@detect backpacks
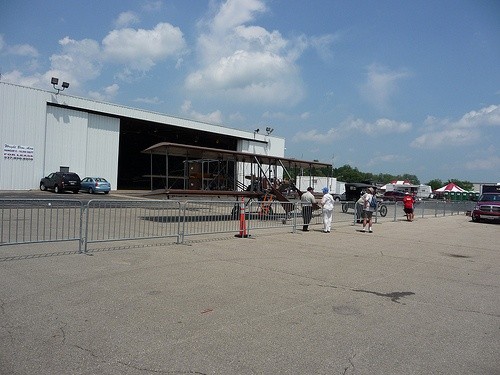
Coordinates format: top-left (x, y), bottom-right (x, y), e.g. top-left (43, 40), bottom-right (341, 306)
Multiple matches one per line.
top-left (370, 197), bottom-right (377, 207)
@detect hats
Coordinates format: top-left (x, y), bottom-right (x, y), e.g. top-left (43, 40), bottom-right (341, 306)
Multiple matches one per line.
top-left (405, 192), bottom-right (409, 195)
top-left (368, 188), bottom-right (374, 194)
top-left (322, 188), bottom-right (328, 193)
top-left (307, 187), bottom-right (313, 191)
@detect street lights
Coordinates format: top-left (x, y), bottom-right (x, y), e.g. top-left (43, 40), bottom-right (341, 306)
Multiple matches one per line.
top-left (253, 129), bottom-right (260, 176)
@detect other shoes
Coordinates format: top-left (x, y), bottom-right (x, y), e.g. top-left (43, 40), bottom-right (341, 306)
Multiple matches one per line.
top-left (321, 230), bottom-right (327, 233)
top-left (360, 230), bottom-right (365, 232)
top-left (370, 231), bottom-right (372, 232)
top-left (327, 231), bottom-right (330, 233)
top-left (304, 230), bottom-right (309, 232)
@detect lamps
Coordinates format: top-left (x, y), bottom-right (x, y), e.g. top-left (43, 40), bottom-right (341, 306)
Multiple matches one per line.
top-left (266, 127), bottom-right (270, 133)
top-left (61, 82), bottom-right (70, 90)
top-left (51, 77), bottom-right (59, 88)
top-left (270, 128), bottom-right (274, 133)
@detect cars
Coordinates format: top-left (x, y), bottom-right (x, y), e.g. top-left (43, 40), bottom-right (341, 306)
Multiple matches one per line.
top-left (471, 193), bottom-right (500, 223)
top-left (383, 191), bottom-right (413, 202)
top-left (79, 177), bottom-right (111, 194)
top-left (341, 183), bottom-right (388, 217)
top-left (333, 192), bottom-right (347, 202)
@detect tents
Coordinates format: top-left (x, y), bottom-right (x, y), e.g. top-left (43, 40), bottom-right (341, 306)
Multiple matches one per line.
top-left (434, 182), bottom-right (467, 195)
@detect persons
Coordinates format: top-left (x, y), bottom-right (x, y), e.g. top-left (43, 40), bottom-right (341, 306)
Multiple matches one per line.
top-left (360, 187), bottom-right (376, 232)
top-left (403, 192), bottom-right (415, 222)
top-left (300, 186), bottom-right (319, 231)
top-left (321, 188), bottom-right (334, 233)
top-left (357, 197), bottom-right (364, 222)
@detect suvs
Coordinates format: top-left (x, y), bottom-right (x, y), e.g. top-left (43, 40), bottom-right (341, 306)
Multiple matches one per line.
top-left (40, 172), bottom-right (82, 194)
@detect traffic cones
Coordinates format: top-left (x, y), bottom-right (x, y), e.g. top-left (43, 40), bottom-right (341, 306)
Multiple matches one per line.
top-left (234, 197), bottom-right (252, 238)
